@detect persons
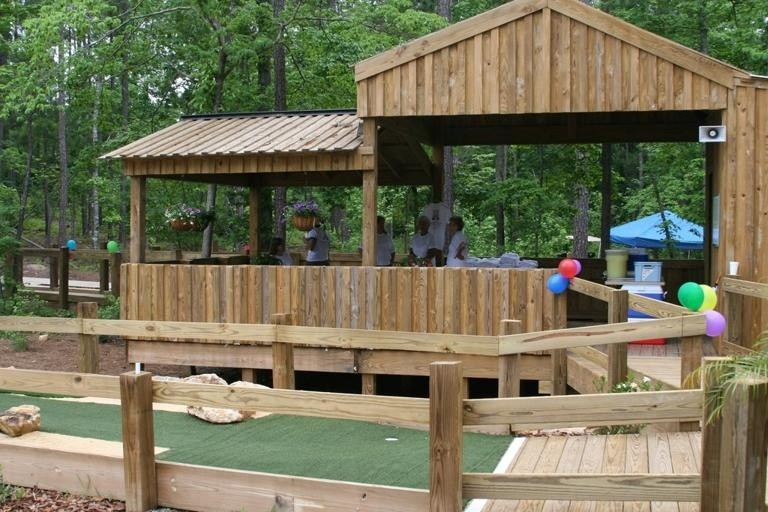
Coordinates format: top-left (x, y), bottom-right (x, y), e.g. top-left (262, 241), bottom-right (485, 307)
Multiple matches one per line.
top-left (269, 215), bottom-right (470, 266)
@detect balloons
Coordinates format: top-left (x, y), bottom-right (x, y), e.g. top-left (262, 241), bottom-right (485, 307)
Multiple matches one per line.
top-left (546, 258), bottom-right (582, 295)
top-left (66, 239), bottom-right (77, 251)
top-left (677, 281), bottom-right (727, 338)
top-left (106, 240), bottom-right (120, 253)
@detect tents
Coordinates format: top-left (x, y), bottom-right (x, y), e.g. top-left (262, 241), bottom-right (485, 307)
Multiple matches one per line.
top-left (608, 209), bottom-right (720, 260)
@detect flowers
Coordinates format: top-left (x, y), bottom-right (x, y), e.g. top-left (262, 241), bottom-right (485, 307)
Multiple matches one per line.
top-left (282, 199), bottom-right (326, 223)
top-left (164, 199), bottom-right (214, 224)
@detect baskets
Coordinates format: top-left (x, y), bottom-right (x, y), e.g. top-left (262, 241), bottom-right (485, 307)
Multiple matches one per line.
top-left (170, 219), bottom-right (199, 231)
top-left (293, 215), bottom-right (319, 231)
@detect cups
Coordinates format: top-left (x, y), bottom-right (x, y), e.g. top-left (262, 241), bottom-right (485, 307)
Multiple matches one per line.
top-left (729, 262), bottom-right (739, 275)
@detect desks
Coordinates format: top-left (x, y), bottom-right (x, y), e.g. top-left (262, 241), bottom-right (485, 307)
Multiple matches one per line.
top-left (603, 270), bottom-right (666, 288)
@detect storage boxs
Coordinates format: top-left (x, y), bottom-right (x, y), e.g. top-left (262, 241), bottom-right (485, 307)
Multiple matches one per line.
top-left (619, 282), bottom-right (667, 317)
top-left (626, 315), bottom-right (668, 345)
top-left (634, 261), bottom-right (666, 284)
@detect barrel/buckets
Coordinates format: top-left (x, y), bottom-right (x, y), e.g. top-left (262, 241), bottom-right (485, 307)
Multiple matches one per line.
top-left (627, 248), bottom-right (648, 276)
top-left (605, 250), bottom-right (628, 278)
